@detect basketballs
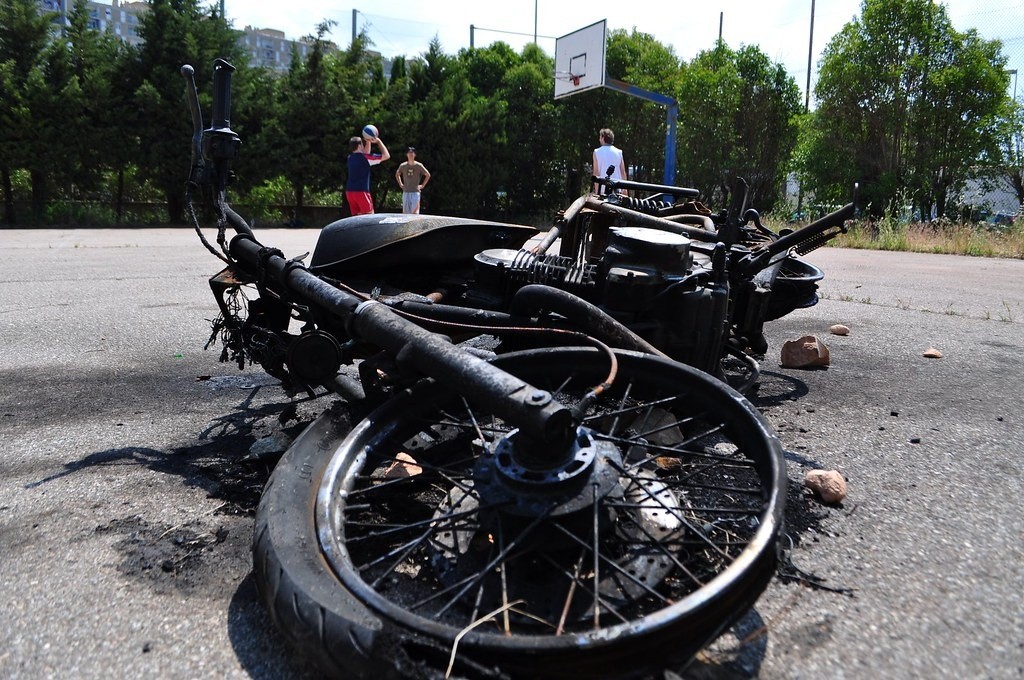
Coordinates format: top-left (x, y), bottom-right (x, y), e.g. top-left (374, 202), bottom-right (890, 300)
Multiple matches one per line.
top-left (362, 124), bottom-right (379, 140)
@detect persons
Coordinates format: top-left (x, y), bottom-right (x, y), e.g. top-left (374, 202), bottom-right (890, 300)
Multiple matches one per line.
top-left (346, 137), bottom-right (390, 215)
top-left (593, 128), bottom-right (627, 197)
top-left (395, 147), bottom-right (430, 215)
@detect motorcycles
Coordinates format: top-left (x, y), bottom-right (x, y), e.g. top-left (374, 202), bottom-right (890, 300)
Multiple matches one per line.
top-left (179, 52), bottom-right (857, 678)
